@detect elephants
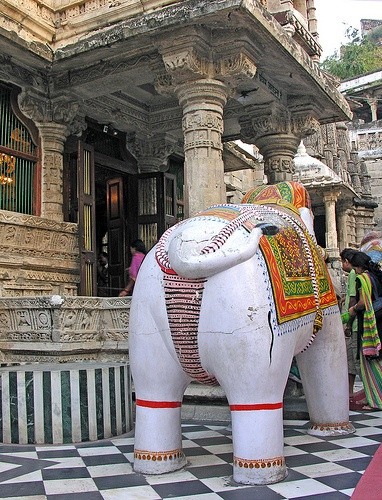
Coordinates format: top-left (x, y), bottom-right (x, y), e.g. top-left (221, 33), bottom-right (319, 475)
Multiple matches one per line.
top-left (128, 180), bottom-right (356, 485)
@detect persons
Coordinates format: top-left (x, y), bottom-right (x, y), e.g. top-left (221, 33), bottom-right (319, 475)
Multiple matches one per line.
top-left (348, 251), bottom-right (382, 411)
top-left (339, 248), bottom-right (369, 405)
top-left (97, 252), bottom-right (109, 297)
top-left (118, 238), bottom-right (146, 297)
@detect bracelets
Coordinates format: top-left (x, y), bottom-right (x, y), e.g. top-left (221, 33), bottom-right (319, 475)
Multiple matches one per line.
top-left (124, 288), bottom-right (129, 293)
top-left (345, 323), bottom-right (352, 330)
top-left (354, 304), bottom-right (359, 313)
